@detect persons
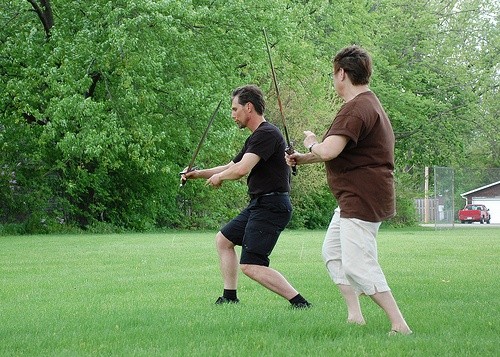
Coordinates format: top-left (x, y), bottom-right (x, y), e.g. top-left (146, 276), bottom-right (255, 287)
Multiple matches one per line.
top-left (181, 83), bottom-right (312, 312)
top-left (285, 47), bottom-right (412, 338)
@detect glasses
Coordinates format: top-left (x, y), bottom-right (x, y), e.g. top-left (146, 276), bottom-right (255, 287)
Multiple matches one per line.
top-left (328, 69), bottom-right (340, 80)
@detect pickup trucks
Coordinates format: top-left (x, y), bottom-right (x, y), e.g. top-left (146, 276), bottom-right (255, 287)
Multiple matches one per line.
top-left (458, 204), bottom-right (491, 224)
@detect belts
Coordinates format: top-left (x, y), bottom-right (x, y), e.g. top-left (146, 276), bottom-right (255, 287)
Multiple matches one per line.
top-left (263, 191), bottom-right (289, 196)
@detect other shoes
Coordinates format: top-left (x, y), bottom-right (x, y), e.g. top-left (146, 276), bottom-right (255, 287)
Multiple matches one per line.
top-left (294, 302), bottom-right (310, 309)
top-left (215, 296), bottom-right (239, 304)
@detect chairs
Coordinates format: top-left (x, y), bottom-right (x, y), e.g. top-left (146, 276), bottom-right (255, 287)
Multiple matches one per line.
top-left (468, 206), bottom-right (472, 209)
top-left (477, 207), bottom-right (481, 210)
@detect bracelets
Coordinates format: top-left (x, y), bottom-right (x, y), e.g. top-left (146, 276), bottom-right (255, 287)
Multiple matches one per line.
top-left (309, 142), bottom-right (317, 152)
